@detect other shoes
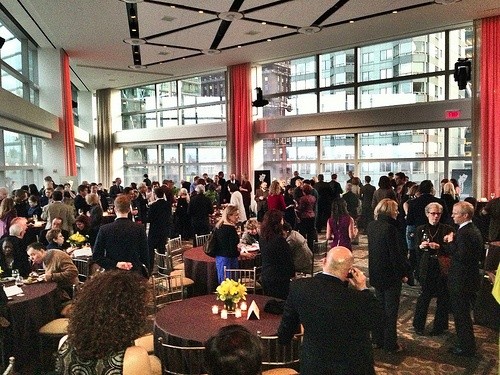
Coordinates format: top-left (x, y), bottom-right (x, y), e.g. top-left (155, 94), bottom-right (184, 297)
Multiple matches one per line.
top-left (449, 345), bottom-right (476, 355)
top-left (384, 343), bottom-right (408, 355)
top-left (414, 328), bottom-right (427, 336)
top-left (432, 324), bottom-right (449, 333)
top-left (371, 342), bottom-right (383, 349)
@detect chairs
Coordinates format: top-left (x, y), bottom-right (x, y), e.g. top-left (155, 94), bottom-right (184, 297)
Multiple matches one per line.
top-left (0, 232), bottom-right (329, 375)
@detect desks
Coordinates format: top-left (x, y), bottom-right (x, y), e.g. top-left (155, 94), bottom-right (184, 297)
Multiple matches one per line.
top-left (183, 244), bottom-right (260, 300)
top-left (0, 281), bottom-right (58, 369)
top-left (153, 294), bottom-right (301, 374)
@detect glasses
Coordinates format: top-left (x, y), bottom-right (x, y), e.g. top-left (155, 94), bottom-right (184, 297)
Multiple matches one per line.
top-left (428, 212), bottom-right (443, 216)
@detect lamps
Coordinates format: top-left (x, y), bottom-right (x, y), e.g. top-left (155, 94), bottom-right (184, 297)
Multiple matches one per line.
top-left (252, 87), bottom-right (269, 107)
top-left (454, 58), bottom-right (472, 89)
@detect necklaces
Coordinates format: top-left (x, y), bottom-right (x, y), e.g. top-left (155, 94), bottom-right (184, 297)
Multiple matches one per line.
top-left (428, 227), bottom-right (439, 241)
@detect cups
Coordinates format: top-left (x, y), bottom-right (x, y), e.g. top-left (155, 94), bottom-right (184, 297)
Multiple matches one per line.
top-left (33, 215), bottom-right (38, 224)
top-left (12, 268), bottom-right (18, 276)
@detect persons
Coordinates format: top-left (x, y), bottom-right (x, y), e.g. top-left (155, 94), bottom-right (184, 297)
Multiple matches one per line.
top-left (485, 262), bottom-right (500, 305)
top-left (0, 170), bottom-right (500, 316)
top-left (278, 246), bottom-right (387, 375)
top-left (201, 324), bottom-right (301, 375)
top-left (411, 201), bottom-right (456, 336)
top-left (52, 269), bottom-right (163, 375)
top-left (367, 198), bottom-right (414, 356)
top-left (427, 201), bottom-right (484, 356)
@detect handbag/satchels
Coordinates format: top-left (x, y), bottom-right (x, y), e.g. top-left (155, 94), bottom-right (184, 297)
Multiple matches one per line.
top-left (203, 228), bottom-right (220, 257)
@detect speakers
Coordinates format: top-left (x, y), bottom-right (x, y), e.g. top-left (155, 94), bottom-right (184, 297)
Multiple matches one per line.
top-left (254, 170), bottom-right (270, 195)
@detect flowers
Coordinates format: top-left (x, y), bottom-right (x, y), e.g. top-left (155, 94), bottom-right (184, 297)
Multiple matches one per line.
top-left (213, 278), bottom-right (248, 305)
